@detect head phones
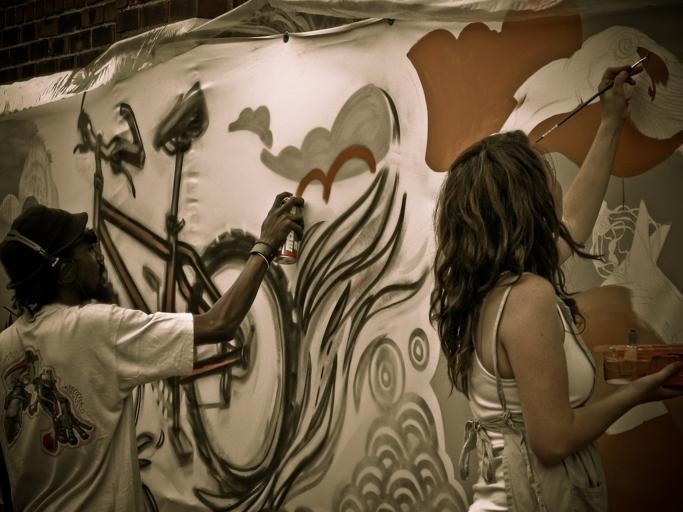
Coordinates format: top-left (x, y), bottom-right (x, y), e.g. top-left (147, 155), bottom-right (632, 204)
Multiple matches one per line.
top-left (4, 230), bottom-right (79, 289)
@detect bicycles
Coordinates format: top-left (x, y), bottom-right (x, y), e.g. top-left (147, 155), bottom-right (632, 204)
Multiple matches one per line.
top-left (73, 81), bottom-right (302, 494)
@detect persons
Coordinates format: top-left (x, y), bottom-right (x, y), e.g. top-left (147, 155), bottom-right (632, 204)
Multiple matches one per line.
top-left (428, 65), bottom-right (681, 511)
top-left (0, 191), bottom-right (306, 512)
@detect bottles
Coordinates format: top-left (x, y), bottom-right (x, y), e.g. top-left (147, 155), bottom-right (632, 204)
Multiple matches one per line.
top-left (273, 196), bottom-right (301, 264)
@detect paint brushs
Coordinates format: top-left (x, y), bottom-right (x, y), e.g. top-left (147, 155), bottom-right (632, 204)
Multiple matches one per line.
top-left (535, 51), bottom-right (650, 143)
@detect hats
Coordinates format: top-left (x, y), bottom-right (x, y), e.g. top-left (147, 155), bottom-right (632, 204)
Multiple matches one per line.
top-left (0, 204), bottom-right (88, 290)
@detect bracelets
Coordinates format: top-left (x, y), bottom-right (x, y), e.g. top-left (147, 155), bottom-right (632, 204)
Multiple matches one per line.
top-left (257, 253), bottom-right (270, 271)
top-left (249, 243), bottom-right (275, 265)
top-left (254, 240), bottom-right (274, 250)
